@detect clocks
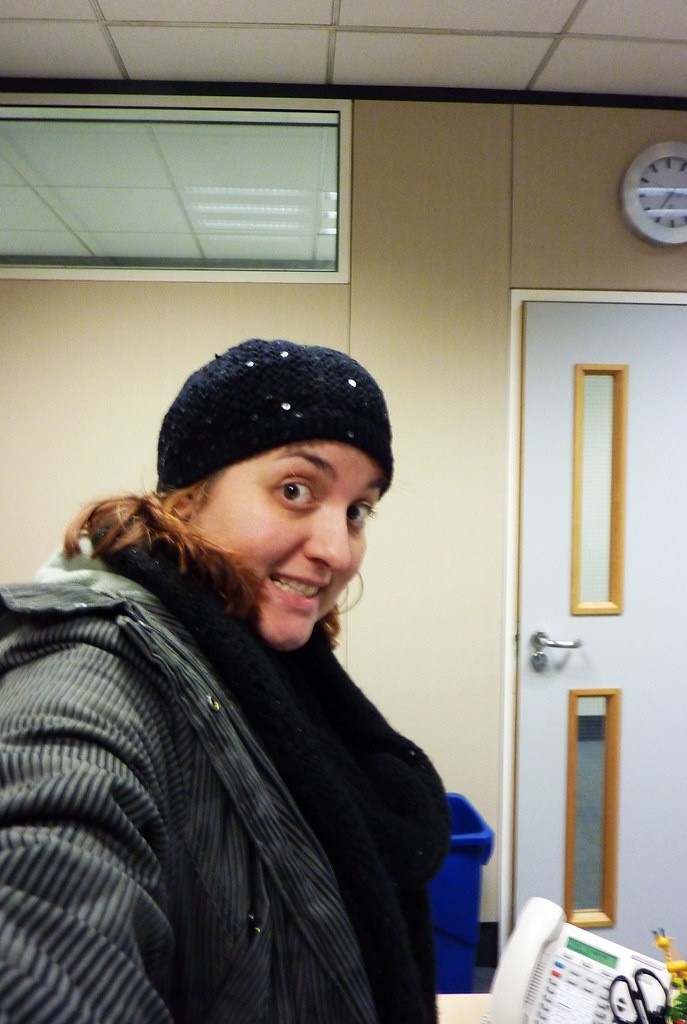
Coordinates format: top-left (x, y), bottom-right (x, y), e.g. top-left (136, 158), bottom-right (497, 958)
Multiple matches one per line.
top-left (619, 141), bottom-right (686, 247)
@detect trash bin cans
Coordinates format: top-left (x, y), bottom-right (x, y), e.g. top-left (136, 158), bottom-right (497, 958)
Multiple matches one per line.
top-left (427, 793), bottom-right (496, 993)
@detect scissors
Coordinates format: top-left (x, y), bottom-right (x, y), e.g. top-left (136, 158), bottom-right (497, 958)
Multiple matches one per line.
top-left (609, 967), bottom-right (670, 1024)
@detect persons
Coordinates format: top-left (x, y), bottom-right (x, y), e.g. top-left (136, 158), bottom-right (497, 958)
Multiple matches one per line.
top-left (0, 338), bottom-right (451, 1024)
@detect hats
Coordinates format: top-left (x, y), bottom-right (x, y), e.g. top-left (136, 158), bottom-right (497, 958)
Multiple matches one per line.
top-left (158, 339), bottom-right (394, 502)
